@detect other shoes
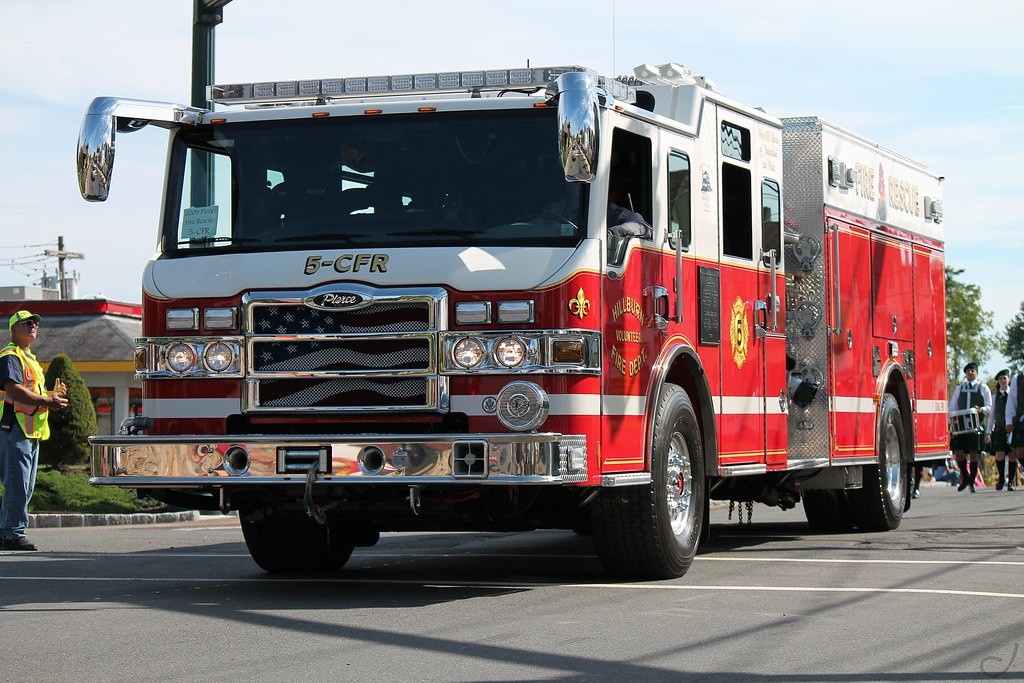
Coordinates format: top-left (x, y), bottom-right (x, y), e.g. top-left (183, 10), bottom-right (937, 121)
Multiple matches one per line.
top-left (912, 488), bottom-right (920, 498)
top-left (0, 537), bottom-right (38, 551)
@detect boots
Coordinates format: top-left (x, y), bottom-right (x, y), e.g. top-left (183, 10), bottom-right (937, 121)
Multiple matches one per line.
top-left (969, 461), bottom-right (978, 492)
top-left (957, 459), bottom-right (970, 491)
top-left (1007, 461), bottom-right (1017, 491)
top-left (995, 458), bottom-right (1006, 490)
top-left (1018, 458), bottom-right (1024, 467)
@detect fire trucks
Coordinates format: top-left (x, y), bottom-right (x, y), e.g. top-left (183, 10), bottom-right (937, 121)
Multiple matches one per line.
top-left (72, 54), bottom-right (955, 583)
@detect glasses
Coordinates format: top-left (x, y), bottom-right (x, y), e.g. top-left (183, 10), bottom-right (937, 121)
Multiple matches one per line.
top-left (17, 320), bottom-right (39, 328)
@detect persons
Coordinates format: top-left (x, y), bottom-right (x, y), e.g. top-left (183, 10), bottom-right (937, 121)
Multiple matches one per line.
top-left (912, 363), bottom-right (1024, 498)
top-left (518, 167), bottom-right (650, 237)
top-left (0, 310), bottom-right (68, 551)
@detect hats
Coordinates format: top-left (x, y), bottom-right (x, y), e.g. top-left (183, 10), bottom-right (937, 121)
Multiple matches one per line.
top-left (9, 310), bottom-right (42, 329)
top-left (963, 363), bottom-right (979, 373)
top-left (995, 369), bottom-right (1009, 380)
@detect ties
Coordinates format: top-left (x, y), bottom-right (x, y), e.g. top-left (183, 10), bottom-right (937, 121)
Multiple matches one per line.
top-left (969, 383), bottom-right (972, 389)
top-left (1004, 391), bottom-right (1007, 396)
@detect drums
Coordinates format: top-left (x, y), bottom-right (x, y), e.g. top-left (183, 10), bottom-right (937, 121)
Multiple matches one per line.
top-left (950, 408), bottom-right (980, 437)
